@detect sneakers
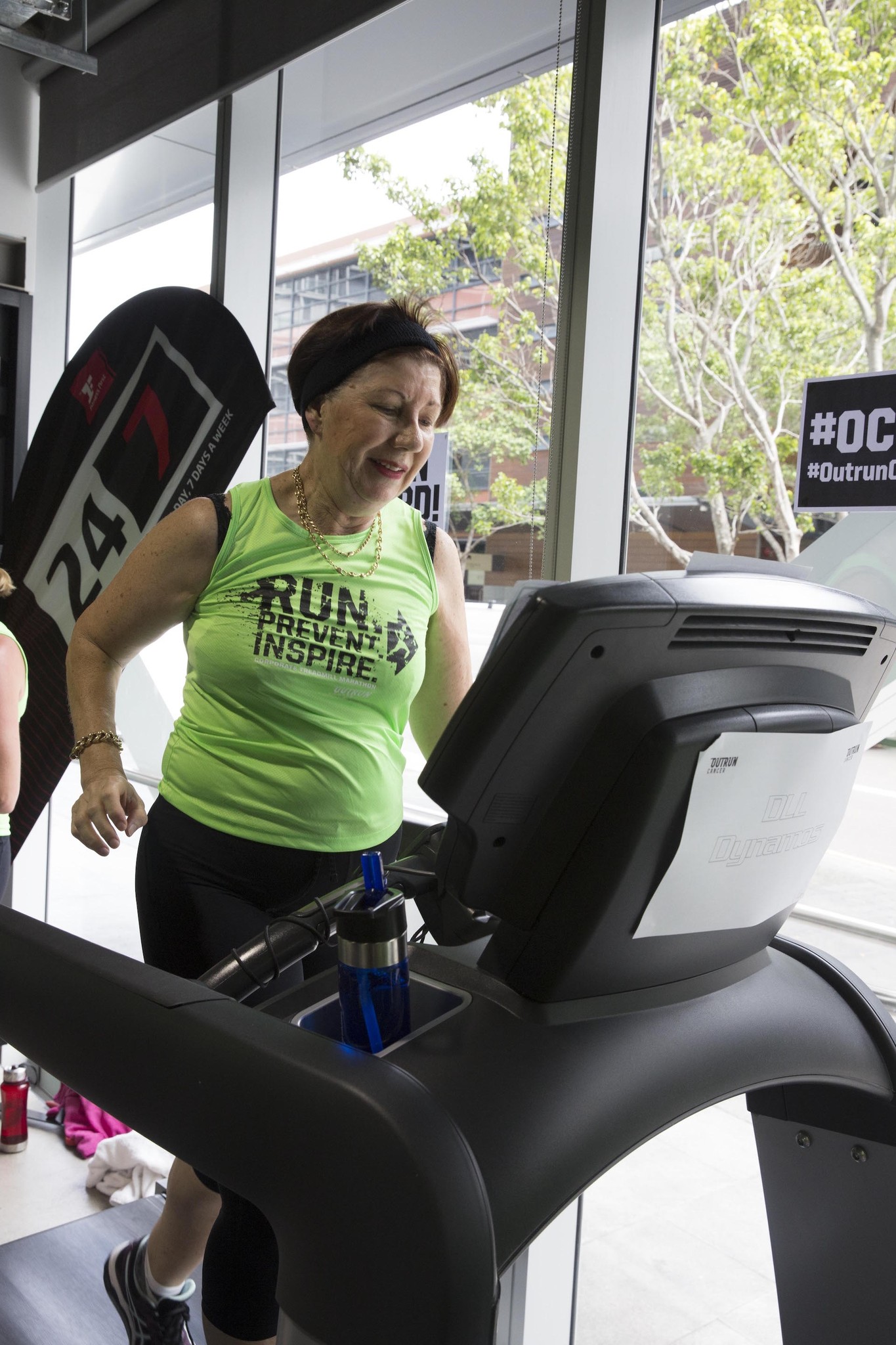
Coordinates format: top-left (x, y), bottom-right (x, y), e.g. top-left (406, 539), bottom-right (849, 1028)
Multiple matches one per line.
top-left (105, 1235), bottom-right (196, 1345)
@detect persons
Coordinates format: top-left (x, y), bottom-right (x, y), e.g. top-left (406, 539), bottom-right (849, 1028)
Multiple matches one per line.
top-left (0, 564), bottom-right (28, 908)
top-left (67, 301), bottom-right (471, 1345)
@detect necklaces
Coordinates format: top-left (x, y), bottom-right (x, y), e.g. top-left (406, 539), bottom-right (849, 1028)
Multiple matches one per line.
top-left (292, 465), bottom-right (382, 577)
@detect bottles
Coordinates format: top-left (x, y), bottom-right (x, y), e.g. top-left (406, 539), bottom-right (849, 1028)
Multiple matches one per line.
top-left (0, 1061), bottom-right (38, 1153)
top-left (334, 850), bottom-right (414, 1054)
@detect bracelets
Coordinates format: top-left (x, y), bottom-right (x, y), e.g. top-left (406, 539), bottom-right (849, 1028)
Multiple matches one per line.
top-left (69, 730), bottom-right (123, 761)
